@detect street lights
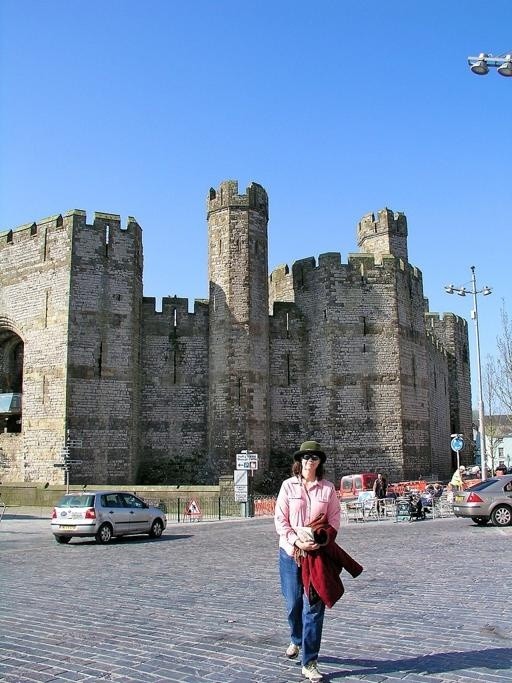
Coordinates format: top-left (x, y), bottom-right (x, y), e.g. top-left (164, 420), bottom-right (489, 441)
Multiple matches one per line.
top-left (443, 265), bottom-right (492, 482)
top-left (450, 431), bottom-right (464, 470)
top-left (466, 52), bottom-right (512, 77)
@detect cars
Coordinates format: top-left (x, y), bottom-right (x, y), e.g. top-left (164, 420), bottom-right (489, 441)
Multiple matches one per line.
top-left (461, 462), bottom-right (511, 481)
top-left (451, 474), bottom-right (512, 526)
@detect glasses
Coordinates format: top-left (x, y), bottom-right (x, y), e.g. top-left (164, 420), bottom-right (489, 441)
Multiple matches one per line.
top-left (301, 454), bottom-right (320, 461)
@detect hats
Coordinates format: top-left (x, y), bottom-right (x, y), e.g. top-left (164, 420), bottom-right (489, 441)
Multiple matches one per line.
top-left (293, 439), bottom-right (328, 465)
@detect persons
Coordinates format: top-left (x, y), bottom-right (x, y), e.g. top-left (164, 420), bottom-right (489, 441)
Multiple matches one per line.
top-left (418, 484), bottom-right (443, 506)
top-left (450, 465), bottom-right (466, 501)
top-left (373, 473), bottom-right (388, 516)
top-left (273, 440), bottom-right (342, 683)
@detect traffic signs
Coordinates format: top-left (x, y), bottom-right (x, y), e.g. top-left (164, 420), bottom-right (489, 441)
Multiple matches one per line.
top-left (53, 436), bottom-right (82, 472)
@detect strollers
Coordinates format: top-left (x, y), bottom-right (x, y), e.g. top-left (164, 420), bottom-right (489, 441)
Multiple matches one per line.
top-left (407, 493), bottom-right (425, 522)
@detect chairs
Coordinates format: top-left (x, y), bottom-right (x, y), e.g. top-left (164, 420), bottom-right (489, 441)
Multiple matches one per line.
top-left (345, 485), bottom-right (457, 524)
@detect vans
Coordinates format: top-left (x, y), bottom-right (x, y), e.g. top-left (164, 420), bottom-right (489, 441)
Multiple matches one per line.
top-left (339, 473), bottom-right (378, 499)
top-left (50, 489), bottom-right (167, 544)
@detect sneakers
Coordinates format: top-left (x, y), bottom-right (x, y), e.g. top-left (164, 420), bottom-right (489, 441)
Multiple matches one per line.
top-left (299, 659), bottom-right (326, 683)
top-left (285, 641), bottom-right (302, 659)
